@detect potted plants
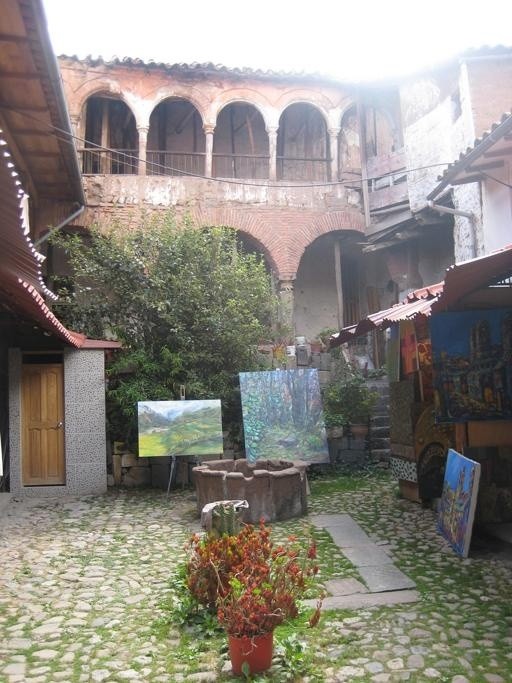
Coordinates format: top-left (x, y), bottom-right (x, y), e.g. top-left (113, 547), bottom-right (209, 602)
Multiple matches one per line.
top-left (319, 371), bottom-right (380, 443)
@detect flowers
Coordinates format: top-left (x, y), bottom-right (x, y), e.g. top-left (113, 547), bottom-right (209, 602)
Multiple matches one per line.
top-left (183, 515), bottom-right (328, 637)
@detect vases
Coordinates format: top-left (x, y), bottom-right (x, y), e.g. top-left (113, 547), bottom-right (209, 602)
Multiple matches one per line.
top-left (226, 628), bottom-right (274, 674)
